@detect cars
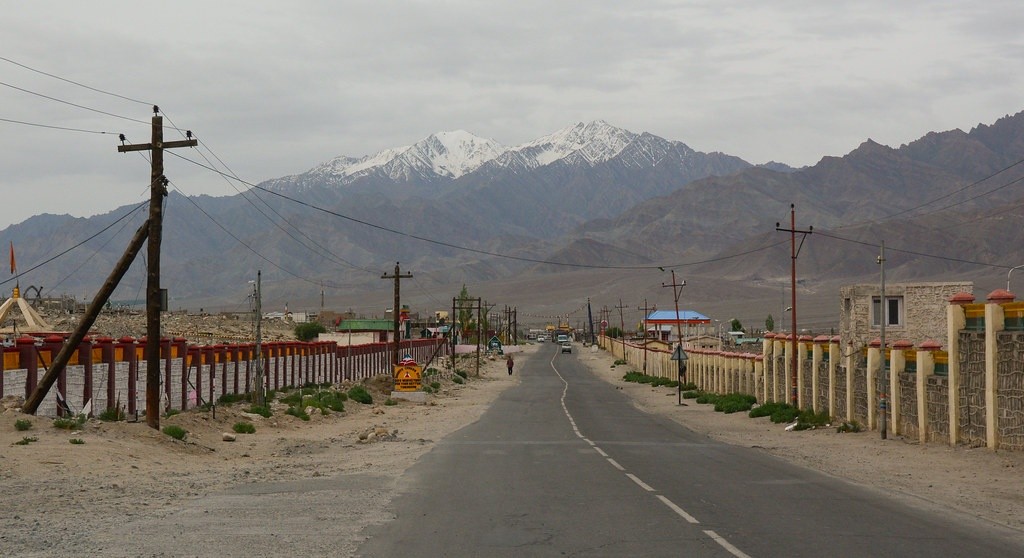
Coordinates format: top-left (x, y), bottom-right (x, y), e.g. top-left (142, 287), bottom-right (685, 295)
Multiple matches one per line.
top-left (538, 335), bottom-right (544, 342)
top-left (557, 335), bottom-right (572, 354)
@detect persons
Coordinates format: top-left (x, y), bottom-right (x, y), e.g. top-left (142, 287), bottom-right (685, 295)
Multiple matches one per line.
top-left (506, 356), bottom-right (514, 375)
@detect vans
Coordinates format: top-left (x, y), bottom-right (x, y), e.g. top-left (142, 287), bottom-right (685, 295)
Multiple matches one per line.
top-left (552, 329), bottom-right (568, 341)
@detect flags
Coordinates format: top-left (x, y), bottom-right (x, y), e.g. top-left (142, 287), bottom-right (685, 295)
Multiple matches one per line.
top-left (9, 243), bottom-right (16, 273)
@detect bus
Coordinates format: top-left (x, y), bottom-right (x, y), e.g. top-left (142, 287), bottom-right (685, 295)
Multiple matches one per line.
top-left (545, 325), bottom-right (556, 333)
top-left (559, 324), bottom-right (570, 332)
